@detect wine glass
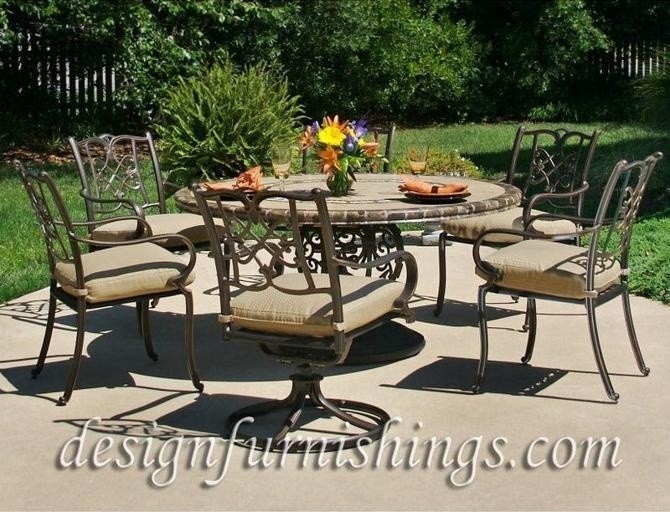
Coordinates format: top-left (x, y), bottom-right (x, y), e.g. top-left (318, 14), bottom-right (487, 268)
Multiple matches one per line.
top-left (406, 144), bottom-right (430, 181)
top-left (271, 142), bottom-right (292, 201)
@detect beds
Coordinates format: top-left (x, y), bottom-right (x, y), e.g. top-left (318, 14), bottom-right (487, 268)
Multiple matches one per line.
top-left (175, 173), bottom-right (522, 366)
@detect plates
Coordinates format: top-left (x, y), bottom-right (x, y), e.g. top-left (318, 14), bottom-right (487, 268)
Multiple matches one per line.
top-left (405, 190), bottom-right (472, 204)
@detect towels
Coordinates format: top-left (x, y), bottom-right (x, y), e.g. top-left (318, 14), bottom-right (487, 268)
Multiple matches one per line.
top-left (234, 166), bottom-right (264, 190)
top-left (400, 178), bottom-right (468, 195)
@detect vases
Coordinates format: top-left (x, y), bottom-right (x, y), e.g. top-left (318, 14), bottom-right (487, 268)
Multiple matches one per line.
top-left (327, 173), bottom-right (352, 196)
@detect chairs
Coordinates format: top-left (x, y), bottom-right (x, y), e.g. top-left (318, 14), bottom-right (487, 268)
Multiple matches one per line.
top-left (13, 160), bottom-right (203, 405)
top-left (192, 185), bottom-right (418, 451)
top-left (69, 132), bottom-right (230, 336)
top-left (433, 127), bottom-right (602, 330)
top-left (471, 152), bottom-right (665, 399)
top-left (302, 123), bottom-right (396, 173)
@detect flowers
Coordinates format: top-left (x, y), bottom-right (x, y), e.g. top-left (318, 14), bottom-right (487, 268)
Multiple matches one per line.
top-left (300, 115), bottom-right (388, 173)
